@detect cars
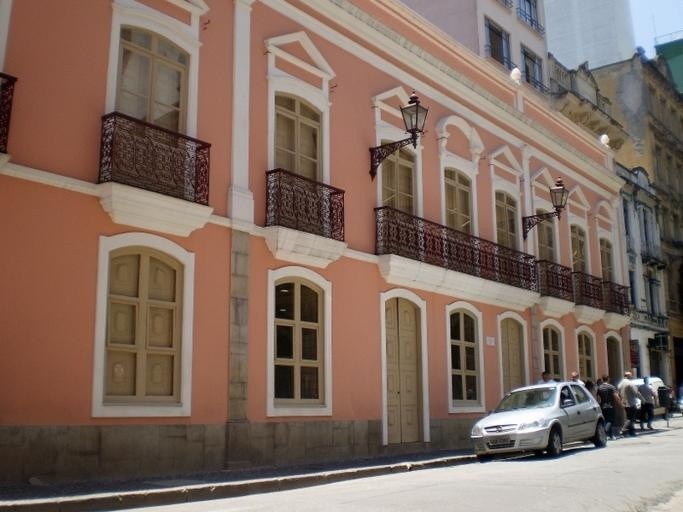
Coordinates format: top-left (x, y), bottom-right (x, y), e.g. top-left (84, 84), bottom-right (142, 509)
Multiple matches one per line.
top-left (470, 381), bottom-right (607, 463)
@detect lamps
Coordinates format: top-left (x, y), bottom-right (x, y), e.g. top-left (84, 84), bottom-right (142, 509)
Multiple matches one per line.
top-left (549, 175), bottom-right (569, 219)
top-left (399, 88), bottom-right (430, 147)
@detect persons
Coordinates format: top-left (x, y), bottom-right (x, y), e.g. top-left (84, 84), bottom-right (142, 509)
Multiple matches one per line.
top-left (571, 372), bottom-right (586, 386)
top-left (597, 375), bottom-right (625, 441)
top-left (638, 377), bottom-right (657, 431)
top-left (597, 379), bottom-right (603, 385)
top-left (585, 381), bottom-right (596, 399)
top-left (537, 371), bottom-right (557, 384)
top-left (618, 372), bottom-right (647, 438)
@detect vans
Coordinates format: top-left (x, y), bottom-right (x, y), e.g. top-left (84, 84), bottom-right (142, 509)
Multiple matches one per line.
top-left (629, 377), bottom-right (664, 409)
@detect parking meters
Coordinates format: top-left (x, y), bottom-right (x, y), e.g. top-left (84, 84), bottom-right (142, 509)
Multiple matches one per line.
top-left (658, 386), bottom-right (670, 429)
